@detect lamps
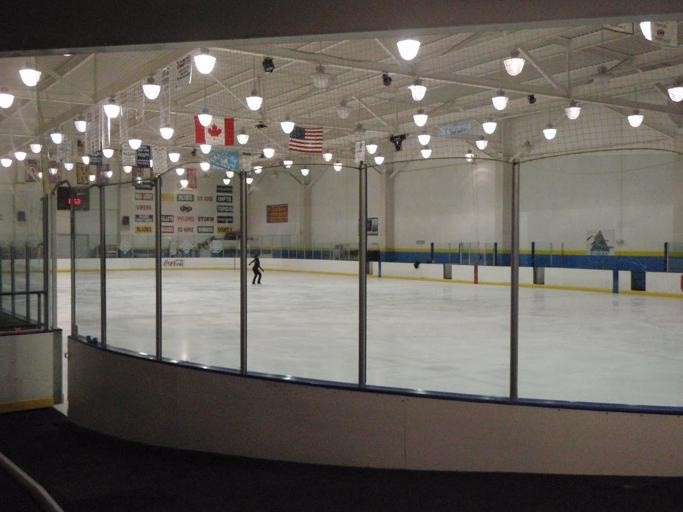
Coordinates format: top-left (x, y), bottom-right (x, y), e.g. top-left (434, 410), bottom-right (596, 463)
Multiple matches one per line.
top-left (0, 21), bottom-right (683, 192)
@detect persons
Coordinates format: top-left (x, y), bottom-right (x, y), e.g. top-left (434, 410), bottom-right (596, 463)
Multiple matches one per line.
top-left (247, 253), bottom-right (265, 284)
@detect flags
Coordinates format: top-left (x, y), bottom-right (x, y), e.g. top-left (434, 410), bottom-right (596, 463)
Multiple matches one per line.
top-left (287, 124), bottom-right (324, 153)
top-left (119, 142), bottom-right (151, 167)
top-left (209, 149), bottom-right (240, 171)
top-left (193, 114), bottom-right (235, 146)
